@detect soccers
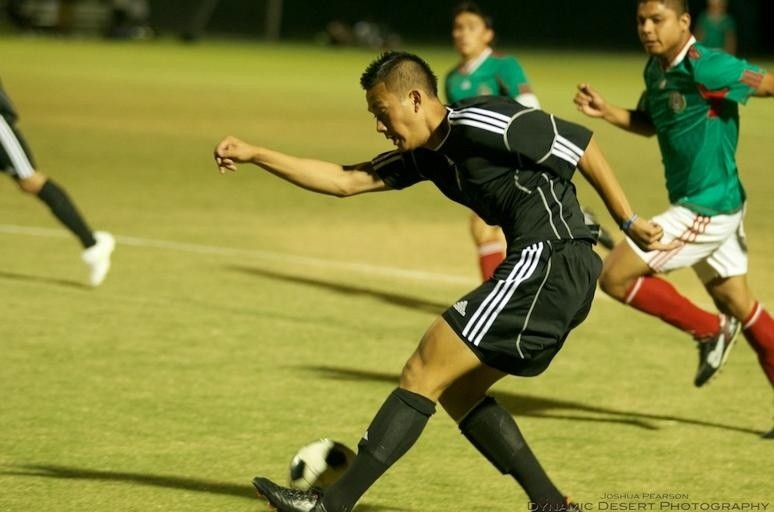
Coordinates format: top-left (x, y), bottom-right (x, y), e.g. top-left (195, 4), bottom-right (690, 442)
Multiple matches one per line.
top-left (290, 438), bottom-right (357, 489)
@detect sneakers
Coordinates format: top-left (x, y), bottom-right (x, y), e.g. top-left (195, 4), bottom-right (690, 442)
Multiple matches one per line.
top-left (694, 313), bottom-right (741, 387)
top-left (83, 230), bottom-right (116, 287)
top-left (581, 208), bottom-right (613, 249)
top-left (251, 476), bottom-right (350, 512)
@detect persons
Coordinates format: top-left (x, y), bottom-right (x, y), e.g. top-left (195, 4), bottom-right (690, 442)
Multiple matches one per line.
top-left (442, 6), bottom-right (541, 282)
top-left (213, 49), bottom-right (682, 512)
top-left (0, 81), bottom-right (115, 287)
top-left (572, 1), bottom-right (773, 441)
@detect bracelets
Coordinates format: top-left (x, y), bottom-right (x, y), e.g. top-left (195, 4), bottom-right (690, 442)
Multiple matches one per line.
top-left (619, 213), bottom-right (637, 232)
